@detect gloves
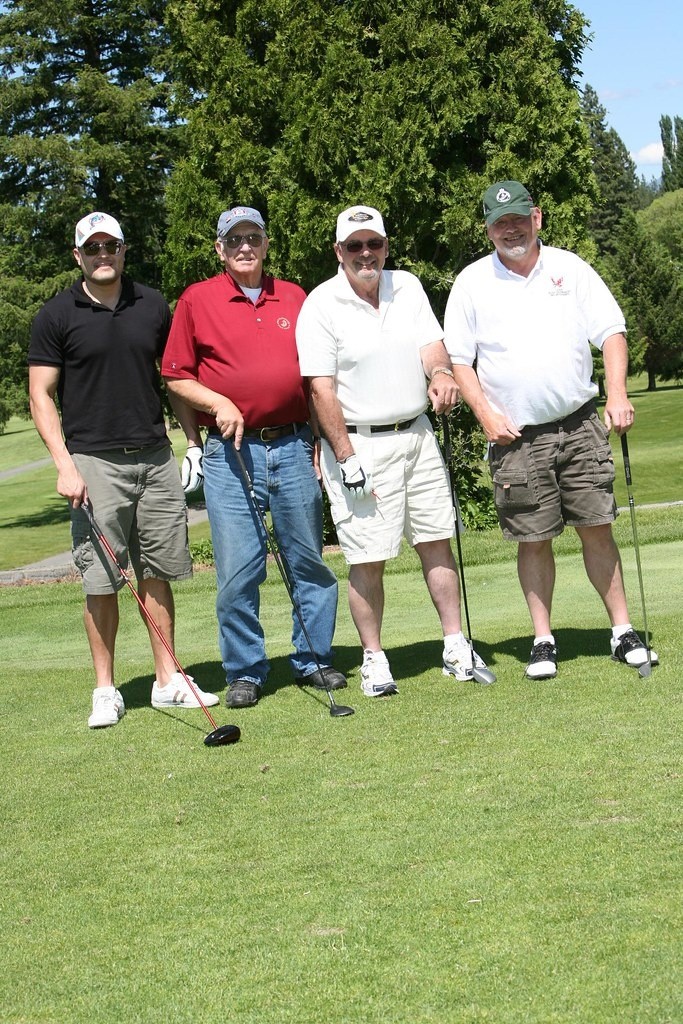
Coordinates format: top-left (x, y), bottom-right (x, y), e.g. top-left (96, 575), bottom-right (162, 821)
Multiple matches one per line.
top-left (180, 446), bottom-right (205, 493)
top-left (336, 453), bottom-right (375, 501)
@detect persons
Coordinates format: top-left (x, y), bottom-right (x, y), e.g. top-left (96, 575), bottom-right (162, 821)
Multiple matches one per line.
top-left (296, 206), bottom-right (486, 697)
top-left (443, 183), bottom-right (661, 679)
top-left (28, 211), bottom-right (220, 731)
top-left (159, 204), bottom-right (349, 707)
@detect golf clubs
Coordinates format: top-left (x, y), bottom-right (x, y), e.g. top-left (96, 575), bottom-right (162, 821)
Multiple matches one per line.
top-left (80, 501), bottom-right (241, 747)
top-left (230, 434), bottom-right (355, 717)
top-left (621, 432), bottom-right (652, 679)
top-left (441, 412), bottom-right (497, 685)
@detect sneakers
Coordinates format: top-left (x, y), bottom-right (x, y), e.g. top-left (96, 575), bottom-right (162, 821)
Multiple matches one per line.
top-left (151, 673), bottom-right (219, 709)
top-left (226, 681), bottom-right (260, 708)
top-left (442, 632), bottom-right (487, 682)
top-left (356, 648), bottom-right (399, 696)
top-left (610, 628), bottom-right (658, 667)
top-left (87, 687), bottom-right (125, 728)
top-left (521, 641), bottom-right (560, 680)
top-left (295, 666), bottom-right (347, 692)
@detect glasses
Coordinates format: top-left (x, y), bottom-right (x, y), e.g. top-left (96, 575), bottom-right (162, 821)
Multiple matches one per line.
top-left (78, 240), bottom-right (124, 257)
top-left (219, 233), bottom-right (265, 249)
top-left (338, 236), bottom-right (385, 253)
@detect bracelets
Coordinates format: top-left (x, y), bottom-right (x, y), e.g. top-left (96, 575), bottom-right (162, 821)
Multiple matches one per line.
top-left (433, 368), bottom-right (455, 377)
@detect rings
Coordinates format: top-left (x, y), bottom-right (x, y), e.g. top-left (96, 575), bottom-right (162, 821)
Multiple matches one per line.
top-left (626, 417), bottom-right (631, 420)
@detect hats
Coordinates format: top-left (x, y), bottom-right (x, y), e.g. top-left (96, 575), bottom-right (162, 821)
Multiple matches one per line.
top-left (74, 212), bottom-right (124, 247)
top-left (217, 206), bottom-right (265, 237)
top-left (336, 205), bottom-right (387, 244)
top-left (483, 181), bottom-right (534, 225)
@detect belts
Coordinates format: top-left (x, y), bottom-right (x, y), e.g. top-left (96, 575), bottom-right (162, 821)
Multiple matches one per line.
top-left (209, 421), bottom-right (306, 442)
top-left (123, 447), bottom-right (143, 455)
top-left (345, 414), bottom-right (419, 433)
